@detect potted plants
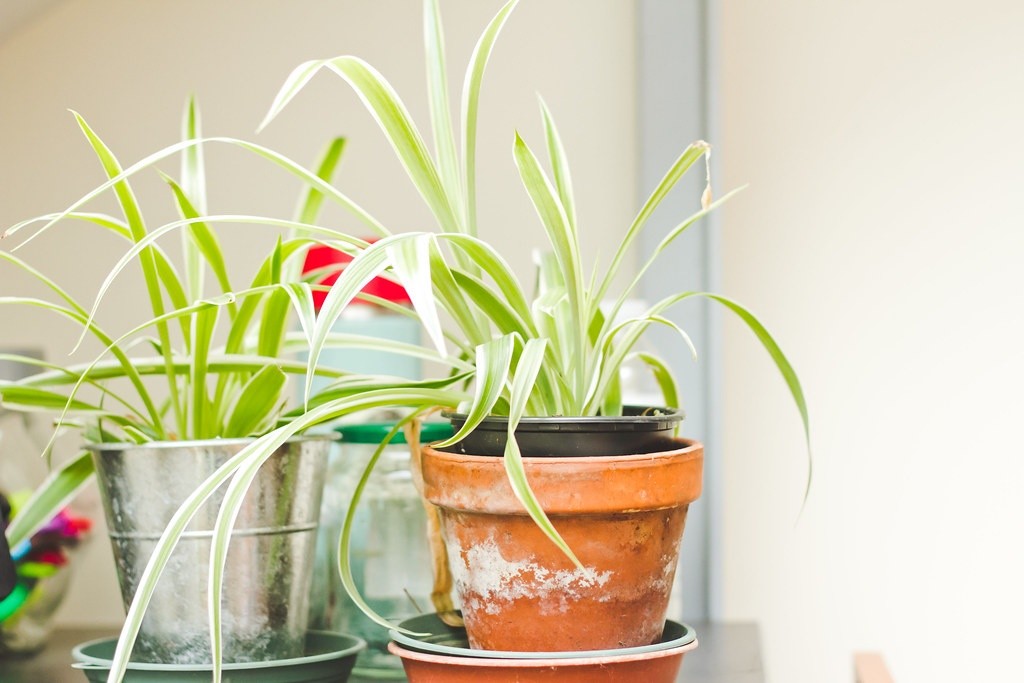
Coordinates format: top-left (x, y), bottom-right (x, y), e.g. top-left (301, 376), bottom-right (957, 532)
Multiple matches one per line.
top-left (0, 1), bottom-right (814, 682)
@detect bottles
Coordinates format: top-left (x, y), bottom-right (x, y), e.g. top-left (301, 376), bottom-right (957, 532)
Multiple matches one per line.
top-left (290, 420), bottom-right (456, 678)
top-left (294, 234), bottom-right (431, 630)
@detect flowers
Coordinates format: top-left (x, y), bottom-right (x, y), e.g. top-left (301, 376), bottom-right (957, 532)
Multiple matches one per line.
top-left (5, 490), bottom-right (91, 569)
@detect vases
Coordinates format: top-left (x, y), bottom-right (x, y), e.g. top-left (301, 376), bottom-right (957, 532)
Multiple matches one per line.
top-left (1, 570), bottom-right (68, 657)
top-left (388, 637), bottom-right (698, 682)
top-left (423, 440), bottom-right (703, 656)
